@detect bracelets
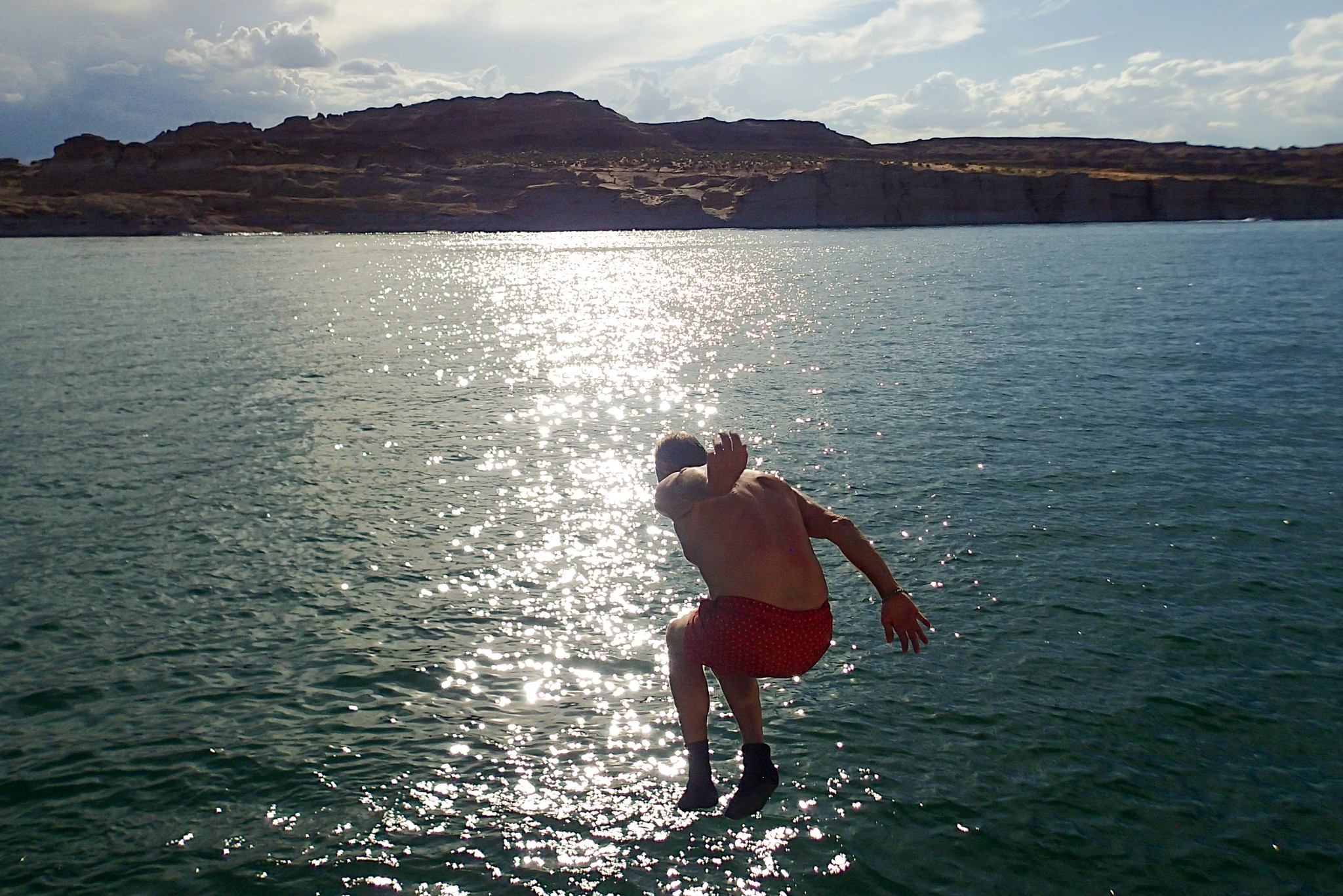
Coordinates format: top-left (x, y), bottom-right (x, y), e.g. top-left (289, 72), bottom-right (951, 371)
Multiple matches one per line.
top-left (882, 589), bottom-right (910, 604)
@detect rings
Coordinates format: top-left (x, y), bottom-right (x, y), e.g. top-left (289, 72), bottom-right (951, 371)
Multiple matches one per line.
top-left (715, 448), bottom-right (723, 453)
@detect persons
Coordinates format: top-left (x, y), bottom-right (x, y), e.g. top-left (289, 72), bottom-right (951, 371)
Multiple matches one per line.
top-left (655, 431), bottom-right (931, 819)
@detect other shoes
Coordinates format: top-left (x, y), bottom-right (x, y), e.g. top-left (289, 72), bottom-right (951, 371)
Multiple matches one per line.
top-left (678, 779), bottom-right (718, 812)
top-left (724, 763), bottom-right (779, 820)
